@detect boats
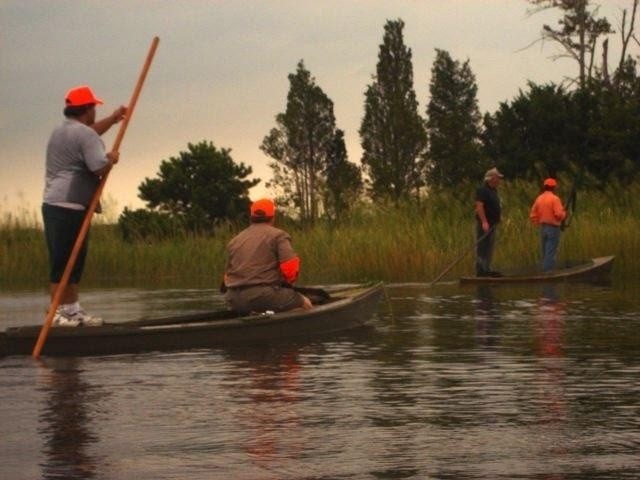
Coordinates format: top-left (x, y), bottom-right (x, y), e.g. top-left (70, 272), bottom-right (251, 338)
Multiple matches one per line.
top-left (1, 278), bottom-right (387, 354)
top-left (459, 255), bottom-right (617, 286)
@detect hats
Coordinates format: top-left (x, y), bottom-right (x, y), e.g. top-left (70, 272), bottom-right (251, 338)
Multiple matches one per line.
top-left (251, 200), bottom-right (274, 217)
top-left (486, 167), bottom-right (503, 178)
top-left (66, 87), bottom-right (103, 106)
top-left (544, 178), bottom-right (556, 187)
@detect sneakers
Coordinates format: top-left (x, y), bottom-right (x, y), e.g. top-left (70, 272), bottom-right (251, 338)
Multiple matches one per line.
top-left (53, 306), bottom-right (104, 327)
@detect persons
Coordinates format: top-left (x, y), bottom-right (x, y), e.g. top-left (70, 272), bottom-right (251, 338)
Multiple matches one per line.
top-left (472, 167), bottom-right (504, 278)
top-left (529, 177), bottom-right (568, 271)
top-left (42, 85), bottom-right (129, 328)
top-left (219, 198), bottom-right (314, 314)
